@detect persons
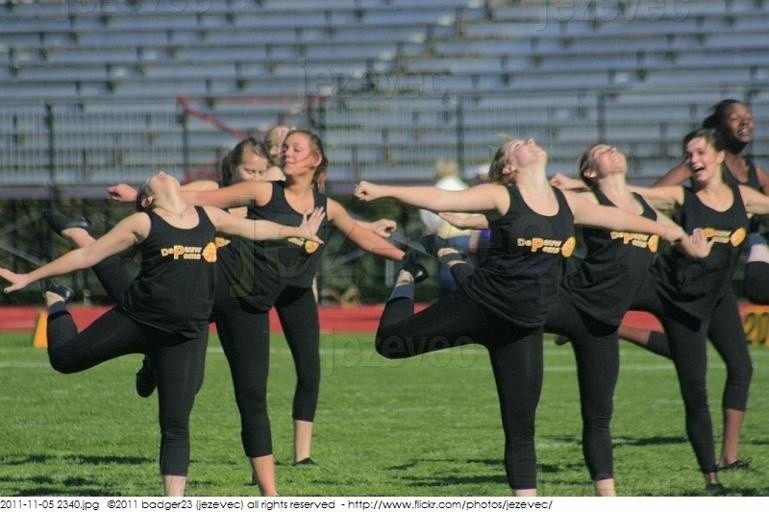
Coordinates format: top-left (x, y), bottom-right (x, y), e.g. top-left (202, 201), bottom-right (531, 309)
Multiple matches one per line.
top-left (0, 99), bottom-right (769, 496)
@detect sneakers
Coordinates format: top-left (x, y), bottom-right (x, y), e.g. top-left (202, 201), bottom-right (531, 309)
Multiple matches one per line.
top-left (39, 275), bottom-right (75, 302)
top-left (136, 357), bottom-right (157, 397)
top-left (717, 459), bottom-right (756, 472)
top-left (41, 209), bottom-right (90, 236)
top-left (400, 252), bottom-right (428, 283)
top-left (420, 234), bottom-right (467, 260)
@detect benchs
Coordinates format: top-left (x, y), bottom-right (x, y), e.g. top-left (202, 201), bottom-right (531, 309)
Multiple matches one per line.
top-left (1, 1), bottom-right (768, 192)
